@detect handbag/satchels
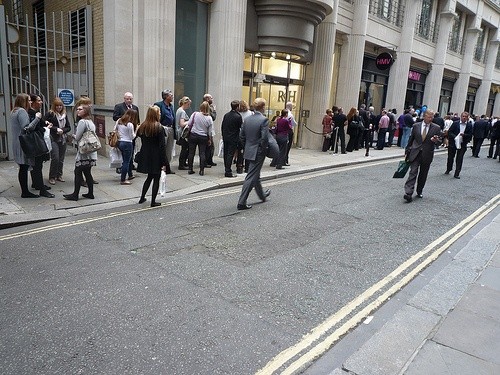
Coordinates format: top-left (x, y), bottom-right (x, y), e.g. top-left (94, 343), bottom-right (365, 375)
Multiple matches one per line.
top-left (217, 138), bottom-right (224, 158)
top-left (350, 115), bottom-right (358, 127)
top-left (18, 126), bottom-right (52, 162)
top-left (454, 133), bottom-right (462, 149)
top-left (105, 119), bottom-right (123, 147)
top-left (76, 120), bottom-right (102, 155)
top-left (159, 169), bottom-right (167, 198)
top-left (391, 161), bottom-right (411, 179)
top-left (108, 147), bottom-right (123, 168)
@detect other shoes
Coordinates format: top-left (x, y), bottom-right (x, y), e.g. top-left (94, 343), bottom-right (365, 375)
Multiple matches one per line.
top-left (176, 161), bottom-right (217, 174)
top-left (62, 193), bottom-right (95, 201)
top-left (82, 181), bottom-right (87, 187)
top-left (227, 159), bottom-right (290, 176)
top-left (139, 197), bottom-right (147, 204)
top-left (165, 169), bottom-right (176, 175)
top-left (119, 175), bottom-right (136, 185)
top-left (444, 169), bottom-right (453, 174)
top-left (453, 174), bottom-right (460, 179)
top-left (49, 176), bottom-right (65, 184)
top-left (403, 194), bottom-right (413, 202)
top-left (261, 189), bottom-right (271, 201)
top-left (31, 182), bottom-right (51, 190)
top-left (417, 192), bottom-right (423, 199)
top-left (237, 204), bottom-right (252, 210)
top-left (20, 190), bottom-right (55, 199)
top-left (151, 201), bottom-right (162, 207)
top-left (93, 179), bottom-right (99, 184)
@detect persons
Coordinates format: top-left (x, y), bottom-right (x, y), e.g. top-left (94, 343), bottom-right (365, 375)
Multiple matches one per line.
top-left (322, 104), bottom-right (398, 156)
top-left (176, 94), bottom-right (217, 176)
top-left (397, 104), bottom-right (427, 149)
top-left (403, 109), bottom-right (442, 202)
top-left (237, 99), bottom-right (279, 210)
top-left (220, 100), bottom-right (255, 178)
top-left (270, 102), bottom-right (297, 171)
top-left (132, 105), bottom-right (167, 207)
top-left (153, 89), bottom-right (176, 174)
top-left (113, 92), bottom-right (141, 185)
top-left (432, 112), bottom-right (460, 149)
top-left (8, 93), bottom-right (71, 198)
top-left (445, 112), bottom-right (472, 178)
top-left (468, 113), bottom-right (500, 163)
top-left (63, 97), bottom-right (100, 201)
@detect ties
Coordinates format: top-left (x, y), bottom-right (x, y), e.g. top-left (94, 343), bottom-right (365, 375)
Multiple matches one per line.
top-left (422, 124), bottom-right (428, 142)
